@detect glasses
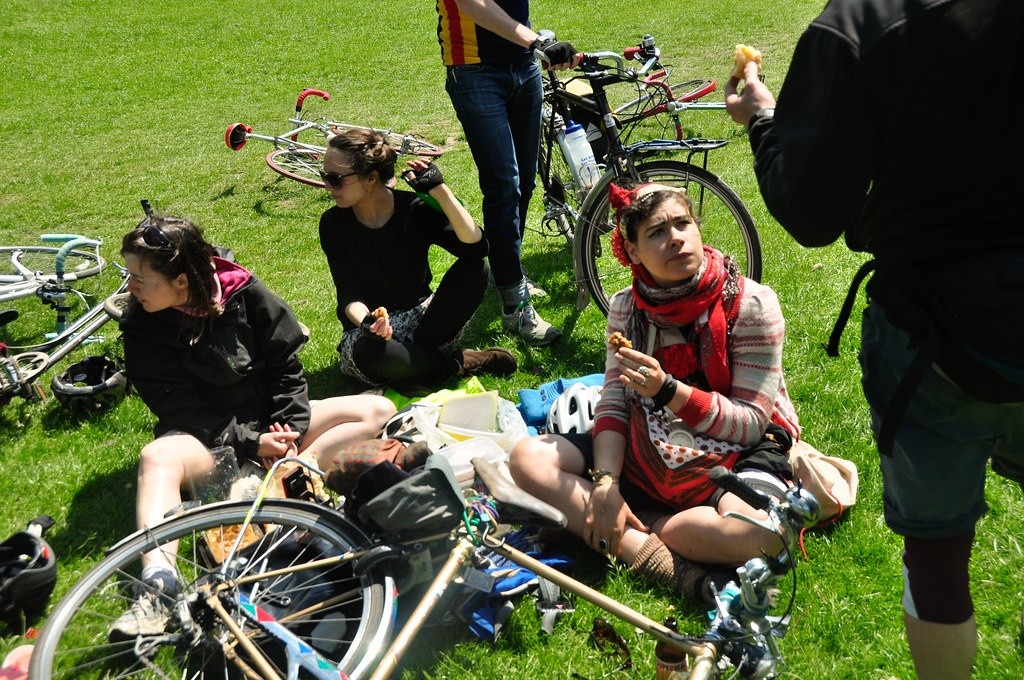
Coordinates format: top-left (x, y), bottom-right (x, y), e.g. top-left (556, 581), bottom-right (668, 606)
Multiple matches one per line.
top-left (142, 227), bottom-right (178, 251)
top-left (319, 171), bottom-right (358, 190)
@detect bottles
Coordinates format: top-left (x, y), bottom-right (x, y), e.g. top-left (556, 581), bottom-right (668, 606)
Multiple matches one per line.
top-left (655, 618), bottom-right (689, 680)
top-left (564, 120), bottom-right (602, 189)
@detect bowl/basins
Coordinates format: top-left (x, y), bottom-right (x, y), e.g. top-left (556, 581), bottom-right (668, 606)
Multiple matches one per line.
top-left (435, 437), bottom-right (508, 491)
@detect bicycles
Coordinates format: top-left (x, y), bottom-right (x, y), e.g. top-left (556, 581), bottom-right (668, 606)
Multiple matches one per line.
top-left (0, 234), bottom-right (130, 404)
top-left (534, 28), bottom-right (763, 316)
top-left (25, 458), bottom-right (822, 680)
top-left (225, 89), bottom-right (444, 189)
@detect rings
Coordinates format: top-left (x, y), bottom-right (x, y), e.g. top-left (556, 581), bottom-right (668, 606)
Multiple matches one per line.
top-left (600, 536), bottom-right (608, 549)
top-left (641, 378), bottom-right (647, 386)
top-left (637, 365), bottom-right (648, 375)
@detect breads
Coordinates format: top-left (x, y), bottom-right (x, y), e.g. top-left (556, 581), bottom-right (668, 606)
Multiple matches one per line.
top-left (608, 331), bottom-right (633, 353)
top-left (732, 44), bottom-right (762, 79)
top-left (373, 306), bottom-right (389, 319)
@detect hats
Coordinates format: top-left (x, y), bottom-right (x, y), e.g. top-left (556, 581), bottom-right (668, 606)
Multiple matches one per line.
top-left (608, 177), bottom-right (685, 243)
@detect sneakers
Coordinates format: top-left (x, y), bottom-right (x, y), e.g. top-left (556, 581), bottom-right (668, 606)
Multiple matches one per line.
top-left (526, 282), bottom-right (551, 307)
top-left (109, 571), bottom-right (184, 649)
top-left (502, 304), bottom-right (562, 346)
top-left (462, 348), bottom-right (518, 378)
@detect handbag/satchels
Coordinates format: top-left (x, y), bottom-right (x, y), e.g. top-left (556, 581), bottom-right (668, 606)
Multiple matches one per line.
top-left (787, 439), bottom-right (859, 523)
top-left (873, 253), bottom-right (1023, 361)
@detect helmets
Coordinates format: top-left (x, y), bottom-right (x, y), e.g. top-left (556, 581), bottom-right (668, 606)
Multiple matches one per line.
top-left (50, 353), bottom-right (128, 415)
top-left (0, 532), bottom-right (57, 639)
top-left (548, 382), bottom-right (605, 437)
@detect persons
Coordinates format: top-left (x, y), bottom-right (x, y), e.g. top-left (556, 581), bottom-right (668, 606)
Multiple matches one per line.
top-left (509, 180), bottom-right (798, 609)
top-left (436, 0), bottom-right (580, 345)
top-left (723, 0), bottom-right (1024, 680)
top-left (319, 127), bottom-right (517, 399)
top-left (109, 198), bottom-right (396, 642)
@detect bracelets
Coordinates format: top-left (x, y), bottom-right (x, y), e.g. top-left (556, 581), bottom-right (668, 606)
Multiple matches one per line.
top-left (587, 467), bottom-right (620, 484)
top-left (651, 374), bottom-right (677, 412)
